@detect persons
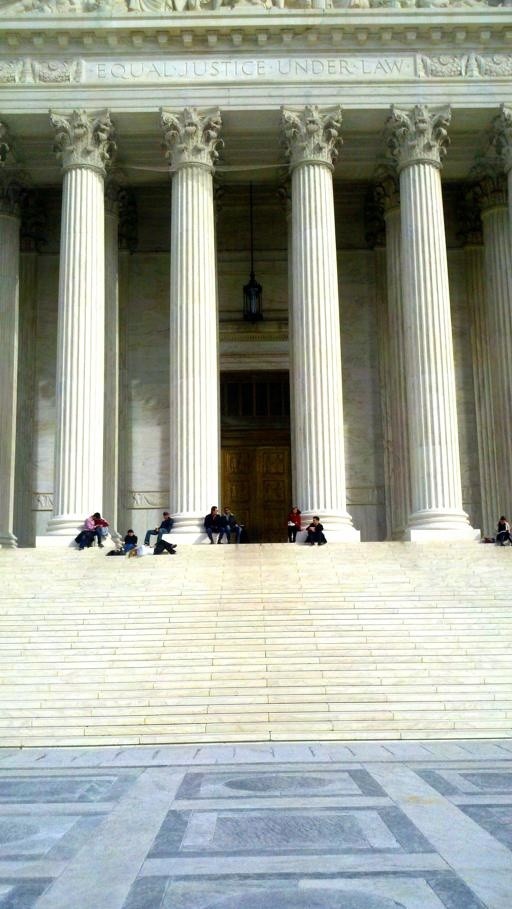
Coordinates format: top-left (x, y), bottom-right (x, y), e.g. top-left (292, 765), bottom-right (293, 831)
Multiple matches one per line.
top-left (83, 512), bottom-right (104, 548)
top-left (222, 505), bottom-right (243, 543)
top-left (203, 505), bottom-right (226, 545)
top-left (122, 528), bottom-right (138, 553)
top-left (496, 514), bottom-right (511, 546)
top-left (304, 515), bottom-right (327, 546)
top-left (127, 539), bottom-right (177, 559)
top-left (286, 505), bottom-right (304, 543)
top-left (143, 511), bottom-right (174, 546)
top-left (74, 530), bottom-right (96, 550)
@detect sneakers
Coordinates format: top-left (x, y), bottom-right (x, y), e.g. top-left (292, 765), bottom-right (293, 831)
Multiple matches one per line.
top-left (170, 543), bottom-right (177, 554)
top-left (79, 544), bottom-right (104, 550)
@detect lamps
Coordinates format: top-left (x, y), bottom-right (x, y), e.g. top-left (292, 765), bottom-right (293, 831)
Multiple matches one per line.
top-left (242, 179), bottom-right (264, 323)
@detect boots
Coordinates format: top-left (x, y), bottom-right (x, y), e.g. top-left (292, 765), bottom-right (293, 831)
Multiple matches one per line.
top-left (209, 537), bottom-right (233, 544)
